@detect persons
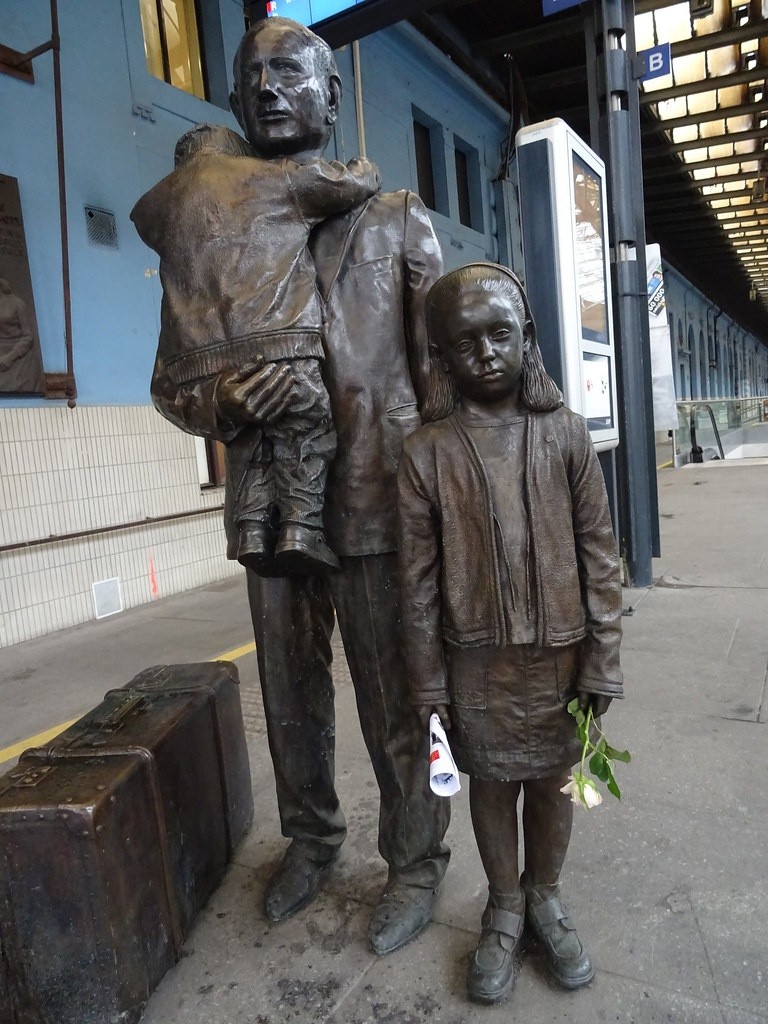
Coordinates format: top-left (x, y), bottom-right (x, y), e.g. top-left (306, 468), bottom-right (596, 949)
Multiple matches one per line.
top-left (127, 19), bottom-right (637, 1004)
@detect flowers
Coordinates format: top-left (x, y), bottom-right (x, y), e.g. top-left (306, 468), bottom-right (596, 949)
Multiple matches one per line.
top-left (558, 697), bottom-right (632, 813)
